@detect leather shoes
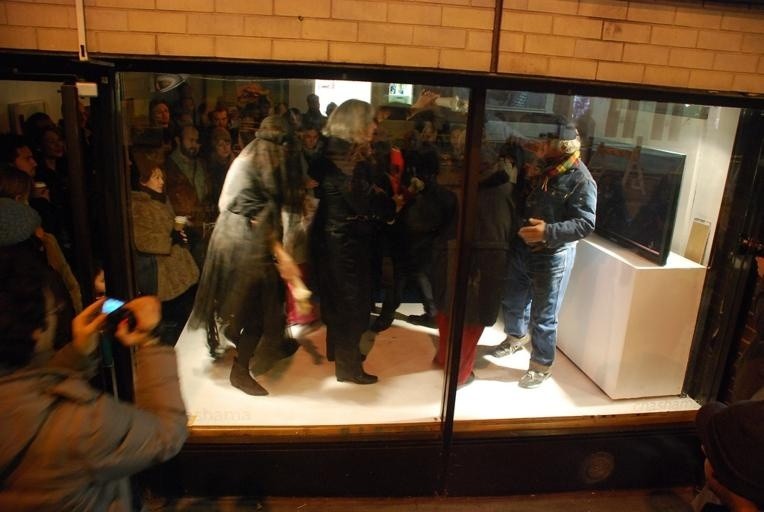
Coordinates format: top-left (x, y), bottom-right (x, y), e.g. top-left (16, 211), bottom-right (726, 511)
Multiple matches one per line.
top-left (336, 370), bottom-right (378, 383)
top-left (327, 350), bottom-right (366, 361)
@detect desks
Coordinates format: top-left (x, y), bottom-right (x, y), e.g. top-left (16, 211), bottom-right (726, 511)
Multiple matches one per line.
top-left (556, 233), bottom-right (705, 400)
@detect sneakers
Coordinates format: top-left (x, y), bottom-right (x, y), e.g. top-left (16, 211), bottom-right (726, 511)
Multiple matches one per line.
top-left (518, 369), bottom-right (553, 389)
top-left (463, 371), bottom-right (474, 384)
top-left (492, 334), bottom-right (531, 357)
top-left (408, 313), bottom-right (439, 329)
top-left (371, 315), bottom-right (395, 332)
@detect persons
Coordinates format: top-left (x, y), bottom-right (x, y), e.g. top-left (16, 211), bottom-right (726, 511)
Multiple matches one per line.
top-left (0, 249), bottom-right (189, 512)
top-left (129, 89), bottom-right (597, 397)
top-left (0, 100), bottom-right (106, 341)
top-left (692, 400), bottom-right (763, 512)
top-left (733, 242), bottom-right (764, 401)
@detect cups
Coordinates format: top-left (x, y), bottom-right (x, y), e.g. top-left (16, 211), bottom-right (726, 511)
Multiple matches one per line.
top-left (31, 181), bottom-right (47, 194)
top-left (173, 215), bottom-right (187, 231)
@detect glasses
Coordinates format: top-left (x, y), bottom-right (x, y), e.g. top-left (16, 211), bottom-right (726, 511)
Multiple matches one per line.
top-left (46, 300), bottom-right (66, 315)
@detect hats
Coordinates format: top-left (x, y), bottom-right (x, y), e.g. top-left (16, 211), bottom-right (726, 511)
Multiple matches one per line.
top-left (1, 197), bottom-right (43, 247)
top-left (539, 125), bottom-right (578, 141)
top-left (210, 127), bottom-right (231, 148)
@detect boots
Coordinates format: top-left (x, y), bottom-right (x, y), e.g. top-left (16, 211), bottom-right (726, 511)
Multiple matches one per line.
top-left (231, 356), bottom-right (268, 396)
top-left (262, 336), bottom-right (299, 361)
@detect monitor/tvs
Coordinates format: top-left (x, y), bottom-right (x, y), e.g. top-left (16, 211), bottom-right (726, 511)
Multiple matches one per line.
top-left (582, 134), bottom-right (686, 267)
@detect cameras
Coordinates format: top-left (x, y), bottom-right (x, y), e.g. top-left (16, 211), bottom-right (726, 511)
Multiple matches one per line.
top-left (95, 295), bottom-right (127, 319)
